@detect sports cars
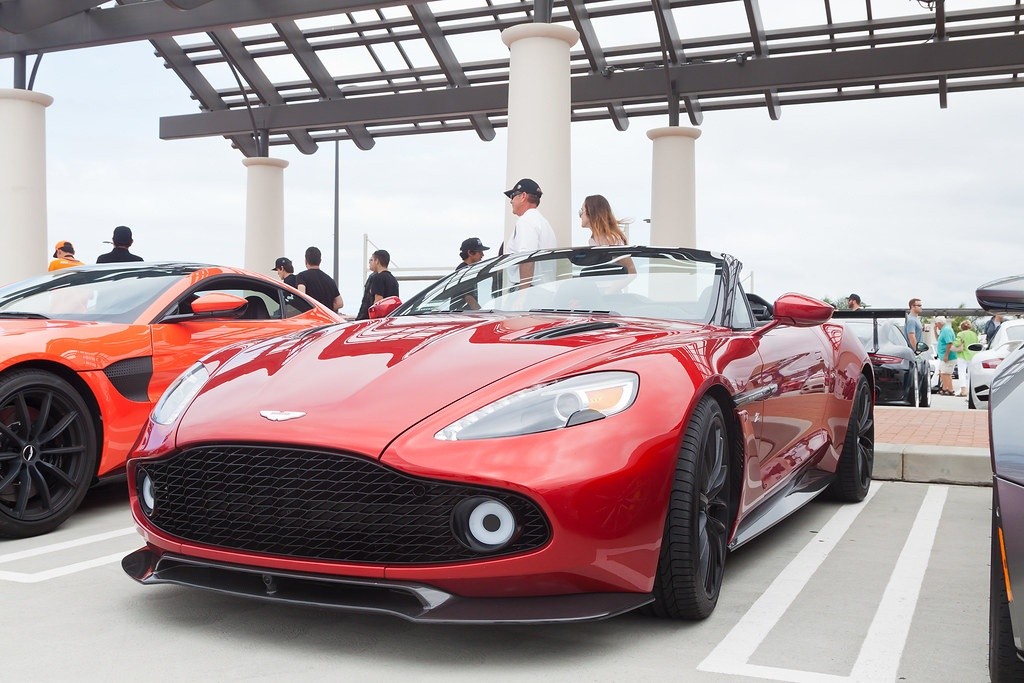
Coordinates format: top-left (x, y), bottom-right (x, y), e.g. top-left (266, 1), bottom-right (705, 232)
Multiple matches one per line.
top-left (120, 244), bottom-right (876, 627)
top-left (0, 261), bottom-right (349, 543)
top-left (975, 272), bottom-right (1024, 683)
top-left (967, 318), bottom-right (1024, 409)
top-left (831, 317), bottom-right (931, 409)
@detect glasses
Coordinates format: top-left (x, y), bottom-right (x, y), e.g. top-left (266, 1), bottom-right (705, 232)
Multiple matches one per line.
top-left (913, 304), bottom-right (922, 308)
top-left (578, 209), bottom-right (587, 217)
top-left (509, 191), bottom-right (522, 200)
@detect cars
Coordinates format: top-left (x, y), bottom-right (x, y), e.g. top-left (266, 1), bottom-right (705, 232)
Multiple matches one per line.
top-left (921, 343), bottom-right (968, 394)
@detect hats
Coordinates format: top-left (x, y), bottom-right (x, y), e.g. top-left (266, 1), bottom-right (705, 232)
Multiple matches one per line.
top-left (113, 226), bottom-right (132, 244)
top-left (462, 238), bottom-right (490, 251)
top-left (272, 257), bottom-right (290, 271)
top-left (53, 241), bottom-right (74, 258)
top-left (846, 294), bottom-right (861, 302)
top-left (503, 179), bottom-right (542, 198)
top-left (934, 316), bottom-right (946, 324)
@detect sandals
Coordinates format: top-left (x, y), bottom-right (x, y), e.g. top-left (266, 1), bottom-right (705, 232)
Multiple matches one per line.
top-left (943, 390), bottom-right (955, 395)
top-left (935, 388), bottom-right (947, 395)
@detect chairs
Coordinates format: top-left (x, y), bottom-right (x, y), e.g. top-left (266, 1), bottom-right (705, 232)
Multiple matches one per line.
top-left (240, 296), bottom-right (270, 319)
top-left (602, 293), bottom-right (650, 319)
top-left (698, 285), bottom-right (759, 323)
top-left (177, 294), bottom-right (201, 315)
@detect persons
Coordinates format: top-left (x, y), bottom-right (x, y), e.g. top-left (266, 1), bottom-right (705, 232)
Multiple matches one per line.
top-left (355, 250), bottom-right (401, 321)
top-left (843, 293), bottom-right (864, 312)
top-left (96, 225), bottom-right (144, 265)
top-left (489, 178), bottom-right (557, 309)
top-left (579, 194), bottom-right (638, 280)
top-left (904, 297), bottom-right (926, 359)
top-left (48, 239), bottom-right (86, 273)
top-left (934, 315), bottom-right (981, 401)
top-left (449, 236), bottom-right (490, 316)
top-left (296, 245), bottom-right (343, 313)
top-left (982, 312), bottom-right (1011, 348)
top-left (272, 257), bottom-right (297, 291)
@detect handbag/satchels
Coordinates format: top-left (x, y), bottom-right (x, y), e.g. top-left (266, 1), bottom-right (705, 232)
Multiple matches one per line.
top-left (950, 342), bottom-right (963, 352)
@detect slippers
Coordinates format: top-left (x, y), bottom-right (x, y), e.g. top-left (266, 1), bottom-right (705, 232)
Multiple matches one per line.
top-left (956, 393), bottom-right (967, 397)
